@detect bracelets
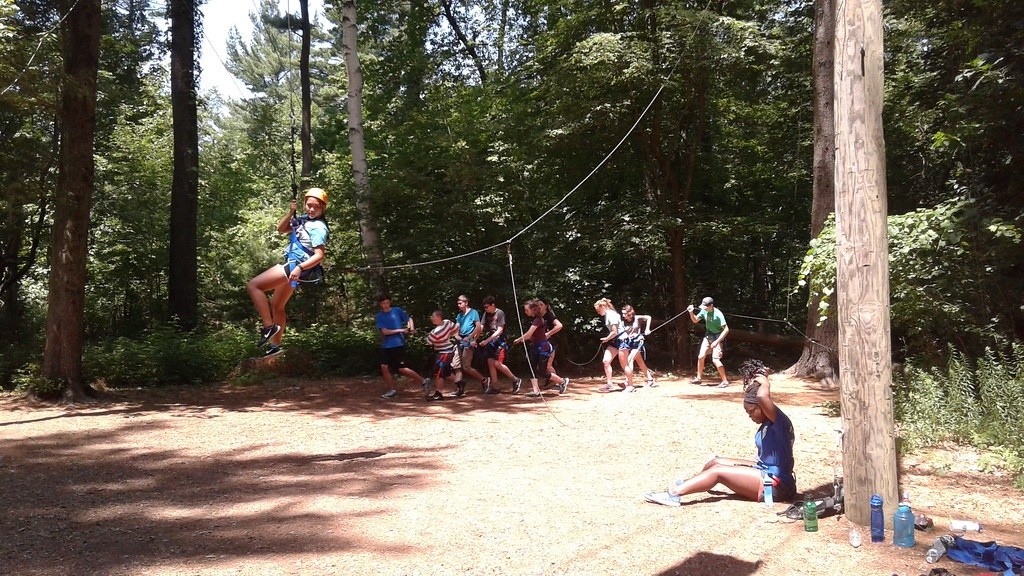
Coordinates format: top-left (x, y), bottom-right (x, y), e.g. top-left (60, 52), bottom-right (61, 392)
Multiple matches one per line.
top-left (298, 264), bottom-right (304, 271)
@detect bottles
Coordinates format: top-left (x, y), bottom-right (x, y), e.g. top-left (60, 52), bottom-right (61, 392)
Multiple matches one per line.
top-left (892, 502), bottom-right (915, 547)
top-left (912, 512), bottom-right (933, 525)
top-left (948, 520), bottom-right (983, 532)
top-left (803, 495), bottom-right (818, 532)
top-left (869, 494), bottom-right (884, 543)
top-left (926, 535), bottom-right (957, 562)
top-left (849, 527), bottom-right (862, 547)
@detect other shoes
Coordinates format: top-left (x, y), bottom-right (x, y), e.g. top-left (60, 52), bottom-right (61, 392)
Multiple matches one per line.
top-left (481, 376), bottom-right (490, 394)
top-left (542, 379), bottom-right (552, 388)
top-left (525, 391), bottom-right (539, 397)
top-left (427, 392), bottom-right (443, 401)
top-left (618, 382), bottom-right (627, 388)
top-left (644, 380), bottom-right (655, 388)
top-left (450, 391), bottom-right (466, 396)
top-left (559, 378), bottom-right (570, 394)
top-left (382, 390), bottom-right (399, 399)
top-left (423, 378), bottom-right (431, 396)
top-left (512, 378), bottom-right (522, 394)
top-left (621, 385), bottom-right (637, 393)
top-left (599, 384), bottom-right (615, 391)
top-left (690, 378), bottom-right (702, 384)
top-left (716, 381), bottom-right (730, 388)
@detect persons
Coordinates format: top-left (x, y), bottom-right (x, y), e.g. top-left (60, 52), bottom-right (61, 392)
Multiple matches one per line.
top-left (374, 295), bottom-right (431, 398)
top-left (617, 305), bottom-right (654, 389)
top-left (688, 296), bottom-right (730, 388)
top-left (593, 297), bottom-right (636, 393)
top-left (469, 296), bottom-right (523, 394)
top-left (451, 295), bottom-right (491, 395)
top-left (424, 311), bottom-right (466, 399)
top-left (245, 188), bottom-right (330, 357)
top-left (643, 358), bottom-right (797, 506)
top-left (513, 298), bottom-right (570, 394)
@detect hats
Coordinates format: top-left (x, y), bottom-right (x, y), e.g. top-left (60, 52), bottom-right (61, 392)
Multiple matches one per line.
top-left (304, 188), bottom-right (328, 205)
top-left (699, 297), bottom-right (714, 310)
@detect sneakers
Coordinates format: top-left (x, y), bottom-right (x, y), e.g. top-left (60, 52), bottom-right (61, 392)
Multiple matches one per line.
top-left (644, 490), bottom-right (681, 507)
top-left (257, 324), bottom-right (281, 348)
top-left (258, 346), bottom-right (284, 360)
